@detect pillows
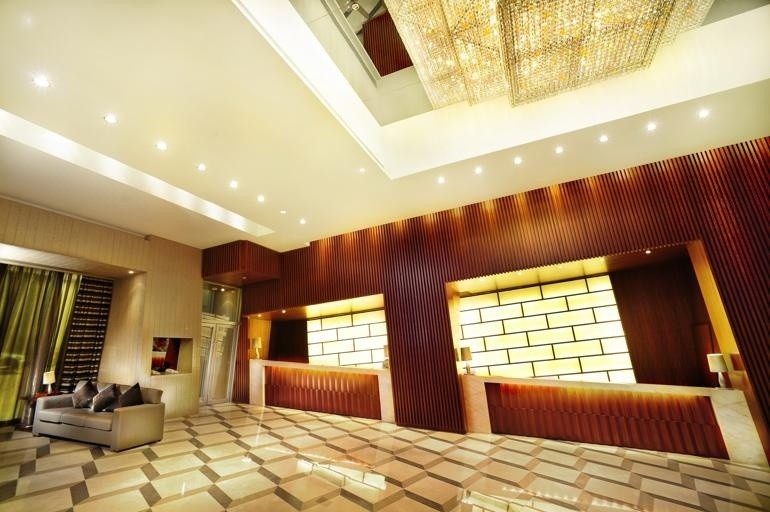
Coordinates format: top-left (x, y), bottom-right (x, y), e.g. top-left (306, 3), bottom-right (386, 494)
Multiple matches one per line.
top-left (71, 380), bottom-right (143, 412)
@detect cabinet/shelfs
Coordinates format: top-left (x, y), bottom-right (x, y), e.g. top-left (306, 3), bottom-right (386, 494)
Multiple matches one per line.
top-left (31, 390), bottom-right (64, 427)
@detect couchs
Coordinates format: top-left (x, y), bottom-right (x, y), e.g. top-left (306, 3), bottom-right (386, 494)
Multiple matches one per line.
top-left (32, 379), bottom-right (165, 453)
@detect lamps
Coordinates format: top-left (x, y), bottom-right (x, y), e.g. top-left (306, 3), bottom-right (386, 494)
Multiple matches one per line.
top-left (251, 336), bottom-right (262, 359)
top-left (707, 353), bottom-right (732, 391)
top-left (43, 371), bottom-right (55, 394)
top-left (461, 346), bottom-right (474, 375)
